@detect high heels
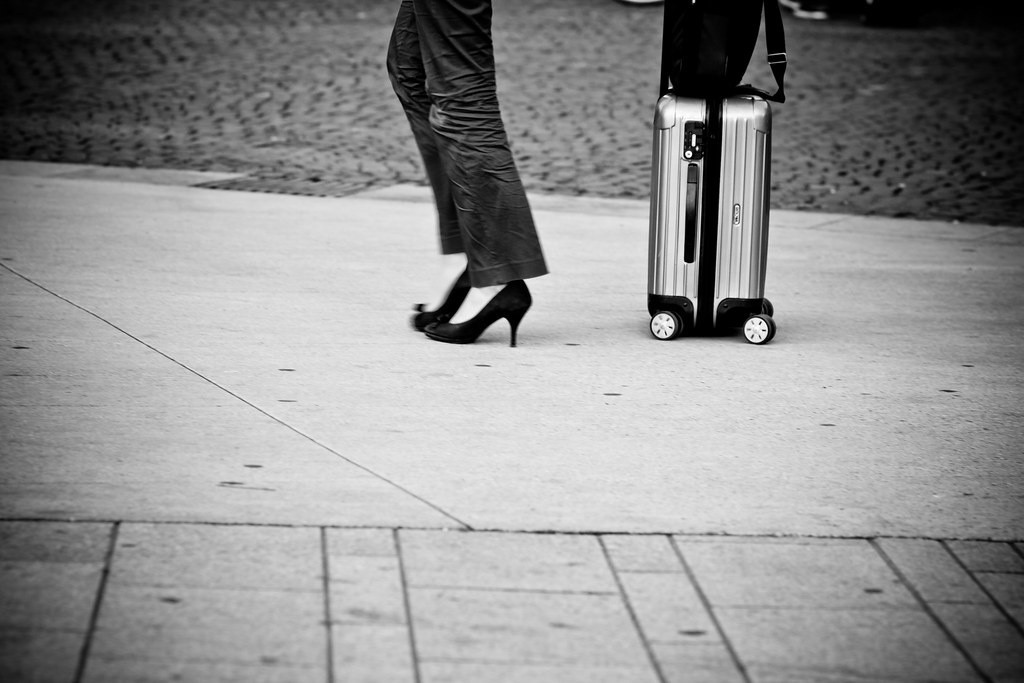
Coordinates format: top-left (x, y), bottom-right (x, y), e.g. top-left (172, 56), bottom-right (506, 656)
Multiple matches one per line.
top-left (414, 262), bottom-right (472, 333)
top-left (424, 276), bottom-right (532, 347)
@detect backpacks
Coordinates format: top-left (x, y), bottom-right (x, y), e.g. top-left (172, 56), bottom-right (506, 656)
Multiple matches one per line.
top-left (666, 0), bottom-right (785, 104)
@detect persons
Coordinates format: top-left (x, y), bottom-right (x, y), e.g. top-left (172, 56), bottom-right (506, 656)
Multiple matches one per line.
top-left (385, 0), bottom-right (550, 346)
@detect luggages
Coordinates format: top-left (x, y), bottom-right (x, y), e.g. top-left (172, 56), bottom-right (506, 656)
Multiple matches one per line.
top-left (645, 0), bottom-right (777, 346)
